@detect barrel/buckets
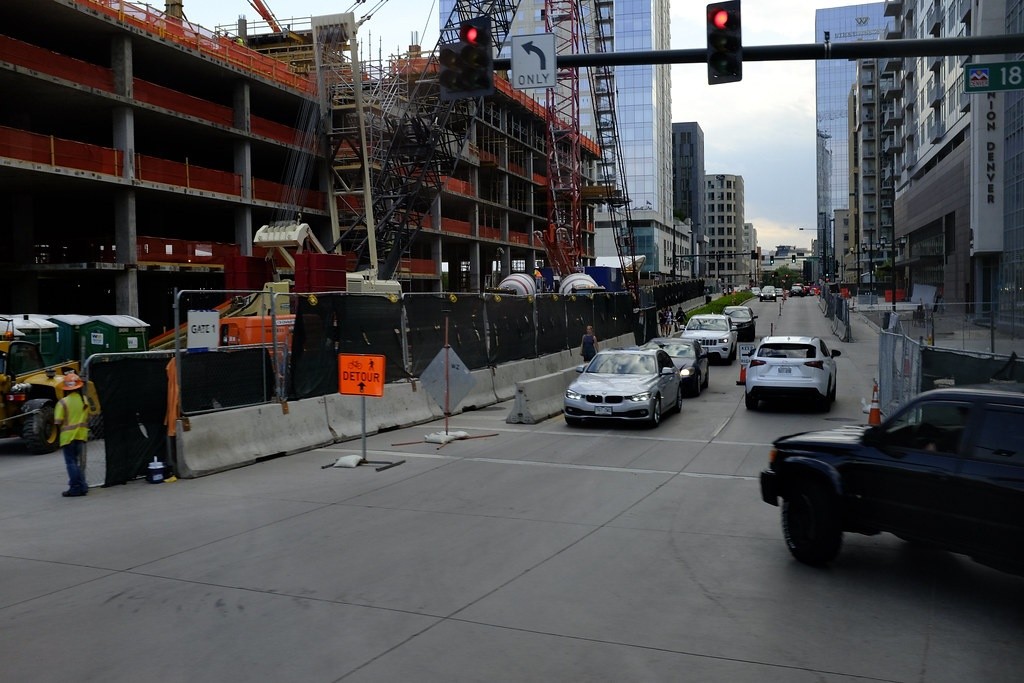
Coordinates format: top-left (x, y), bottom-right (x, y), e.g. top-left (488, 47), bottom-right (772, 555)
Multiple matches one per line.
top-left (147, 456), bottom-right (165, 484)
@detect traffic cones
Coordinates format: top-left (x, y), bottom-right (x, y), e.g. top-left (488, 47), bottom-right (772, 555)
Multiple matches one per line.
top-left (902, 349), bottom-right (910, 377)
top-left (868, 387), bottom-right (880, 426)
top-left (735, 363), bottom-right (747, 385)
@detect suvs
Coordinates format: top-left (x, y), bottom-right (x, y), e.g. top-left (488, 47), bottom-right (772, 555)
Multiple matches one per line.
top-left (760, 286), bottom-right (778, 302)
top-left (760, 376), bottom-right (1024, 583)
top-left (678, 314), bottom-right (738, 365)
top-left (746, 336), bottom-right (843, 410)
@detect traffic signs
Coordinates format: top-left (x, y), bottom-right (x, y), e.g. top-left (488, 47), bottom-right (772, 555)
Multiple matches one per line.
top-left (510, 33), bottom-right (559, 88)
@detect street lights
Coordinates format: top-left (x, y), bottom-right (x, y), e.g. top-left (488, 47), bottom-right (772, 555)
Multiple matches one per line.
top-left (673, 223), bottom-right (701, 281)
top-left (849, 244), bottom-right (880, 293)
top-left (881, 235), bottom-right (907, 312)
top-left (799, 228), bottom-right (826, 277)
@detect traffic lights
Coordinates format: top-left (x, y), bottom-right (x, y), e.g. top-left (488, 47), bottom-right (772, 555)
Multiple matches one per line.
top-left (792, 255), bottom-right (795, 263)
top-left (770, 255), bottom-right (774, 264)
top-left (706, 0), bottom-right (743, 83)
top-left (439, 15), bottom-right (494, 102)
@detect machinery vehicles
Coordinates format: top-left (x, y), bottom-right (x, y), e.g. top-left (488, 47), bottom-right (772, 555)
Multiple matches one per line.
top-left (0, 317), bottom-right (104, 455)
top-left (485, 273), bottom-right (606, 293)
top-left (219, 220), bottom-right (403, 363)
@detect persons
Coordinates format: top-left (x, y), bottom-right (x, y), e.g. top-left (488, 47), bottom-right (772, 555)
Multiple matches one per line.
top-left (533, 268), bottom-right (542, 289)
top-left (580, 325), bottom-right (600, 363)
top-left (658, 306), bottom-right (687, 337)
top-left (703, 288), bottom-right (710, 304)
top-left (54, 373), bottom-right (89, 497)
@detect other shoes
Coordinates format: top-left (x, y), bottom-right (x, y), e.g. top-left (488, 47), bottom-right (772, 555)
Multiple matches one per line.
top-left (63, 487), bottom-right (88, 497)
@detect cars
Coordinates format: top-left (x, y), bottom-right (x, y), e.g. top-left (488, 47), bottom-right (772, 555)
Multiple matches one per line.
top-left (564, 347), bottom-right (684, 427)
top-left (786, 283), bottom-right (818, 296)
top-left (721, 306), bottom-right (759, 341)
top-left (749, 287), bottom-right (783, 296)
top-left (645, 338), bottom-right (709, 398)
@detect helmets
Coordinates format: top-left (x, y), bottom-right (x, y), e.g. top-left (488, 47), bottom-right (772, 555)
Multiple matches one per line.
top-left (60, 373), bottom-right (84, 390)
